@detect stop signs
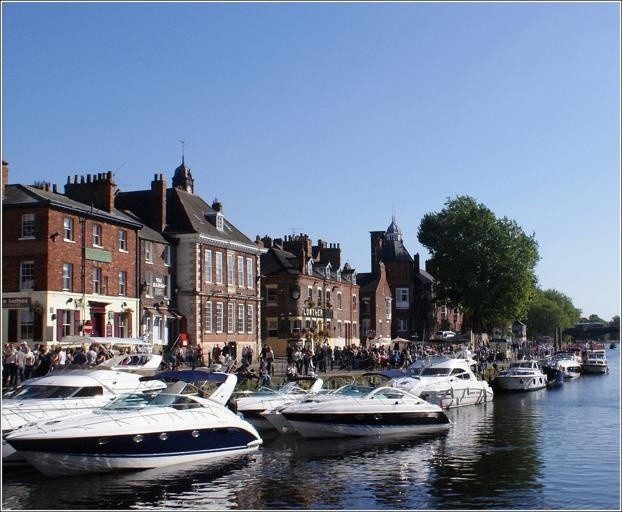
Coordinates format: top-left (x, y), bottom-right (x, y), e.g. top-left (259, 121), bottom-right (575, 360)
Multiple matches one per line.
top-left (84, 319), bottom-right (94, 335)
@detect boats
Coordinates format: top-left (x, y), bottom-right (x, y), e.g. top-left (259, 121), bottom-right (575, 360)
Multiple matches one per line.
top-left (497, 359), bottom-right (549, 392)
top-left (350, 370), bottom-right (406, 393)
top-left (383, 356), bottom-right (495, 412)
top-left (539, 339), bottom-right (615, 383)
top-left (228, 376), bottom-right (344, 417)
top-left (261, 385), bottom-right (379, 438)
top-left (4, 367), bottom-right (264, 479)
top-left (0, 337), bottom-right (169, 443)
top-left (282, 384), bottom-right (452, 440)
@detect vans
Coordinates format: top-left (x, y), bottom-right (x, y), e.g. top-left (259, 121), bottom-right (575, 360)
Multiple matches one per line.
top-left (434, 330), bottom-right (456, 342)
